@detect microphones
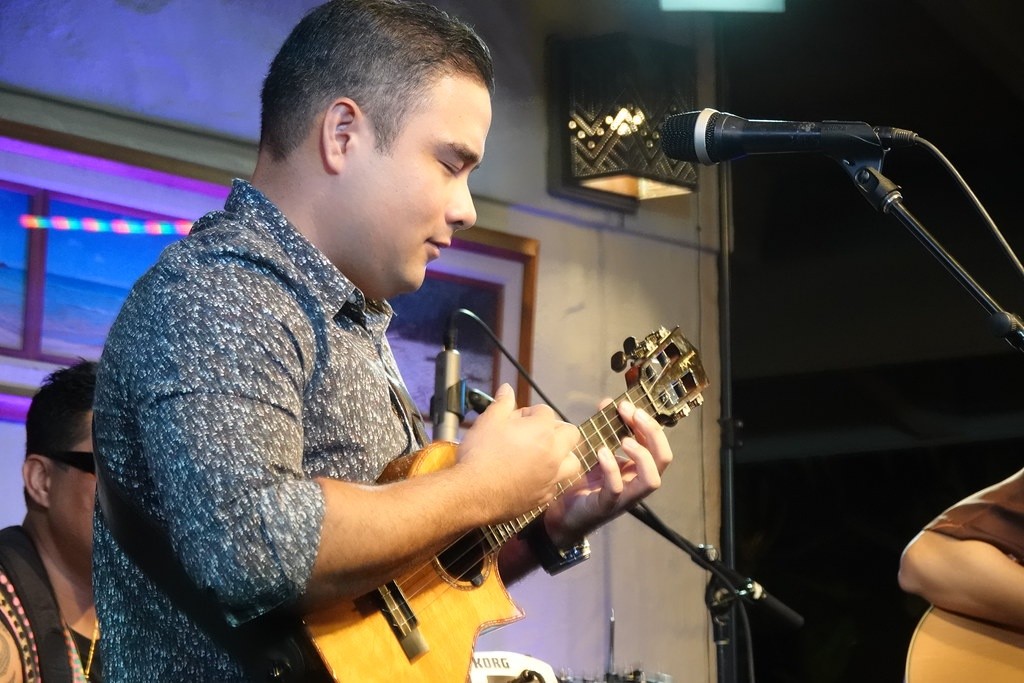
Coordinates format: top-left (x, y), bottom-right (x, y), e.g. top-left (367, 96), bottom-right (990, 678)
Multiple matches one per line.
top-left (660, 107), bottom-right (913, 164)
top-left (435, 349), bottom-right (462, 444)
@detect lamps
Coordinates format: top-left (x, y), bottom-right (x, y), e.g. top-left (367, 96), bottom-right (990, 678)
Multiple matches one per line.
top-left (565, 30), bottom-right (701, 205)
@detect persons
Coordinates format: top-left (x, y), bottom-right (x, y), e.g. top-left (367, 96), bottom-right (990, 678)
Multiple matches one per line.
top-left (0, 351), bottom-right (103, 683)
top-left (90, 2), bottom-right (675, 683)
top-left (898, 466), bottom-right (1024, 633)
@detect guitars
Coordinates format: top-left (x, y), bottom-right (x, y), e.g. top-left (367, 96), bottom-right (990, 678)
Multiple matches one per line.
top-left (901, 605), bottom-right (1024, 683)
top-left (287, 325), bottom-right (711, 683)
